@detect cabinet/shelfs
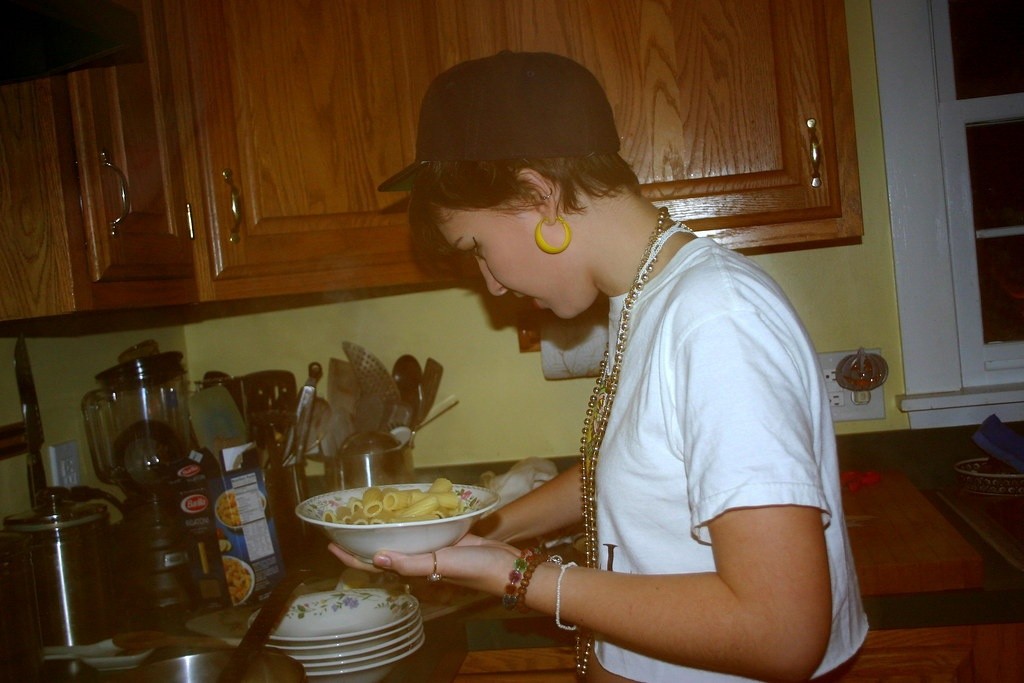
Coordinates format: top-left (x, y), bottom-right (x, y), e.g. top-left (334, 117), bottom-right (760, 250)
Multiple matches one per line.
top-left (0, 0), bottom-right (867, 319)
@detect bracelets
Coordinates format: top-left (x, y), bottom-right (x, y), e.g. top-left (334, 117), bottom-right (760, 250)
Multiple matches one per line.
top-left (548, 555), bottom-right (578, 630)
top-left (502, 546), bottom-right (549, 615)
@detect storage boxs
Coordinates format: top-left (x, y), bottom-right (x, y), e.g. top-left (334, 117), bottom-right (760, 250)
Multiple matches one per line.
top-left (213, 470), bottom-right (285, 607)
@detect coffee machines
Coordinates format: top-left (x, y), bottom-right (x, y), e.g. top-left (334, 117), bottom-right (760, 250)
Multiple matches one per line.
top-left (82, 340), bottom-right (194, 610)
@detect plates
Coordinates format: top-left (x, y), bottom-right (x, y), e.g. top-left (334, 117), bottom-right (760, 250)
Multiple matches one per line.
top-left (222, 555), bottom-right (255, 606)
top-left (35, 639), bottom-right (155, 671)
top-left (214, 488), bottom-right (267, 530)
top-left (248, 588), bottom-right (426, 683)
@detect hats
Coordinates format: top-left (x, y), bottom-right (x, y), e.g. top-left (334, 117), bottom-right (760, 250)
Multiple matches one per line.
top-left (378, 49), bottom-right (621, 192)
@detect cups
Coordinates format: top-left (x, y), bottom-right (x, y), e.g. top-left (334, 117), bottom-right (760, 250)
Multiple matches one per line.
top-left (1, 486), bottom-right (120, 651)
top-left (0, 533), bottom-right (47, 683)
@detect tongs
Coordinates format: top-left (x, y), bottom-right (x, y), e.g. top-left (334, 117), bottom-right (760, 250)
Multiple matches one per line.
top-left (283, 363), bottom-right (322, 467)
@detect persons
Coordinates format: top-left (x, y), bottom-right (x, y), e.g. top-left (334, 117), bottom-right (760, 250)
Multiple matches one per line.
top-left (327, 50), bottom-right (870, 683)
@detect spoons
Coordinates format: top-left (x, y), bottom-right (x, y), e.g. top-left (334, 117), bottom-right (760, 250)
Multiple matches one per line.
top-left (113, 631), bottom-right (236, 649)
top-left (381, 395), bottom-right (459, 451)
top-left (388, 353), bottom-right (423, 410)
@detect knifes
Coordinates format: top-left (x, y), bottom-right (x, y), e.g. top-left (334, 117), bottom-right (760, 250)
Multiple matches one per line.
top-left (15, 334), bottom-right (48, 510)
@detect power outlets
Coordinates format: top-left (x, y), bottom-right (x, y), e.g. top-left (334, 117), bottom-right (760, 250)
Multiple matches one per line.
top-left (815, 349), bottom-right (886, 423)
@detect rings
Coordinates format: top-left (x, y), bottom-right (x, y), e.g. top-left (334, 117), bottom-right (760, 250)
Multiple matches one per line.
top-left (426, 552), bottom-right (441, 584)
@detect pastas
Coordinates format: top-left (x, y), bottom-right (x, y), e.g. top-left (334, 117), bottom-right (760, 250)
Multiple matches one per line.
top-left (323, 477), bottom-right (468, 525)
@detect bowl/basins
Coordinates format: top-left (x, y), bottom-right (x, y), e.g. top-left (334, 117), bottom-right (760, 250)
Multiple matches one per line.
top-left (954, 458), bottom-right (1024, 511)
top-left (295, 484), bottom-right (501, 565)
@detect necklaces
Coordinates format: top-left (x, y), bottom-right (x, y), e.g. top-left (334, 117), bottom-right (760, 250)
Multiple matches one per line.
top-left (574, 205), bottom-right (670, 679)
